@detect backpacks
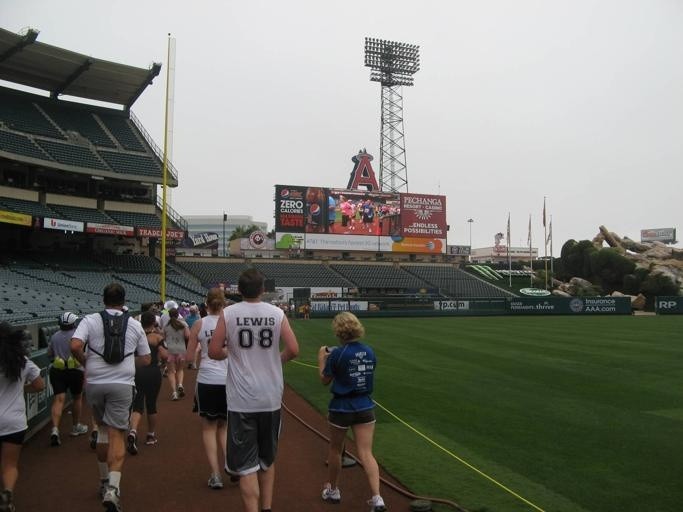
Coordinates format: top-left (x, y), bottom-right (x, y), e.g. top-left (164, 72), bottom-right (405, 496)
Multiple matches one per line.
top-left (89, 310), bottom-right (134, 364)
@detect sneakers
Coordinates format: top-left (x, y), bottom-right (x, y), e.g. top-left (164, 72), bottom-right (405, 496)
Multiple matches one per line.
top-left (103, 487), bottom-right (124, 512)
top-left (50, 424), bottom-right (159, 456)
top-left (168, 391), bottom-right (178, 402)
top-left (0, 488), bottom-right (18, 512)
top-left (178, 385), bottom-right (184, 397)
top-left (367, 497), bottom-right (387, 512)
top-left (322, 489), bottom-right (342, 501)
top-left (208, 477), bottom-right (225, 488)
top-left (163, 367), bottom-right (168, 378)
top-left (97, 479), bottom-right (110, 495)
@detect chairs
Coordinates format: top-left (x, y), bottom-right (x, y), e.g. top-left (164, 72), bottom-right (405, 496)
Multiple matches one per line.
top-left (0, 85), bottom-right (174, 179)
top-left (0, 195), bottom-right (183, 232)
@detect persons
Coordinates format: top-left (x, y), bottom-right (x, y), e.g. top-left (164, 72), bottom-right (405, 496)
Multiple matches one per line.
top-left (207, 269), bottom-right (298, 512)
top-left (70, 282), bottom-right (151, 512)
top-left (185, 288), bottom-right (239, 488)
top-left (47, 313), bottom-right (88, 447)
top-left (274, 302), bottom-right (311, 320)
top-left (318, 312), bottom-right (385, 512)
top-left (329, 195), bottom-right (400, 231)
top-left (1, 320), bottom-right (44, 511)
top-left (123, 300), bottom-right (207, 454)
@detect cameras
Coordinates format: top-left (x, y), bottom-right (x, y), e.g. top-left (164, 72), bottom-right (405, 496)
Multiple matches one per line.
top-left (154, 300), bottom-right (163, 307)
top-left (326, 345), bottom-right (339, 353)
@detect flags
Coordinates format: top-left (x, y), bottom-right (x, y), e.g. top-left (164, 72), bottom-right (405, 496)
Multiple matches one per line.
top-left (547, 224), bottom-right (551, 245)
top-left (543, 206), bottom-right (546, 226)
top-left (526, 220), bottom-right (531, 245)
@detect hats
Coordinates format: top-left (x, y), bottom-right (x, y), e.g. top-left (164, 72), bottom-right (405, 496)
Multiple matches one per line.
top-left (62, 312), bottom-right (79, 325)
top-left (164, 301), bottom-right (179, 312)
